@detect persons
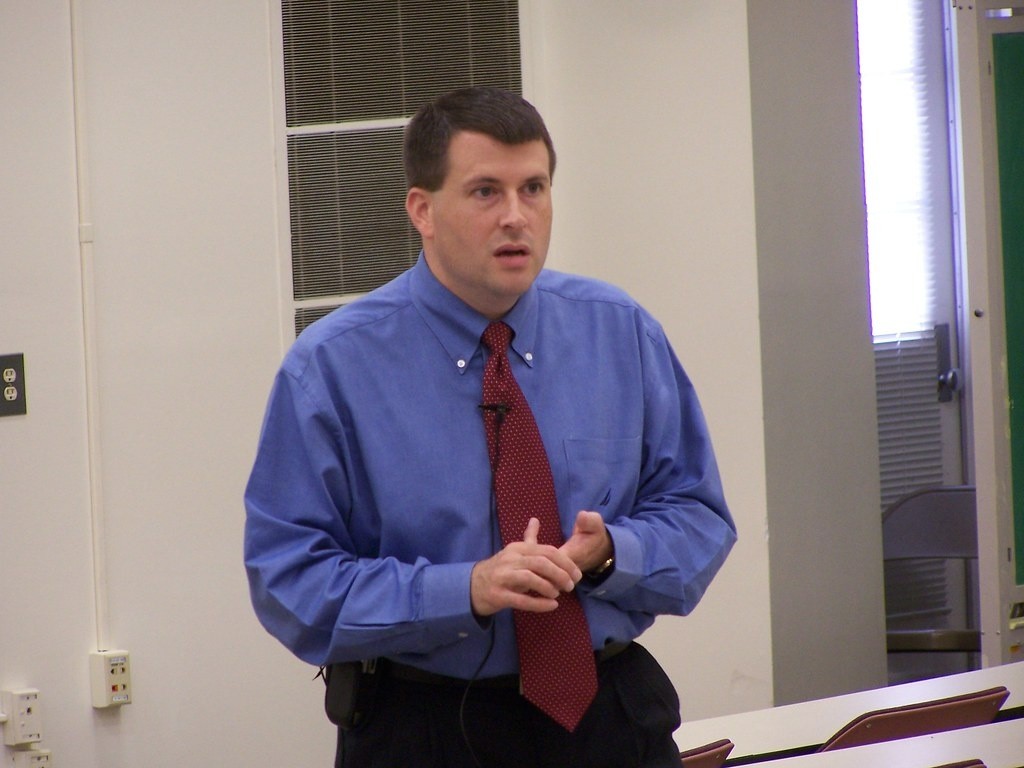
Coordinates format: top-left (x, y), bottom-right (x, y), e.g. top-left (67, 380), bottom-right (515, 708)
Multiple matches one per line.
top-left (243, 89), bottom-right (739, 768)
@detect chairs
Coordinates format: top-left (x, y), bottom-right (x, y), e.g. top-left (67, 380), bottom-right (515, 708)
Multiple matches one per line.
top-left (680, 736), bottom-right (736, 768)
top-left (881, 484), bottom-right (982, 685)
top-left (816, 685), bottom-right (1012, 755)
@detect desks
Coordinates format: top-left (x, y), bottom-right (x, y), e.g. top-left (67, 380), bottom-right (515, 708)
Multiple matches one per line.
top-left (669, 660), bottom-right (1024, 760)
top-left (727, 717), bottom-right (1024, 768)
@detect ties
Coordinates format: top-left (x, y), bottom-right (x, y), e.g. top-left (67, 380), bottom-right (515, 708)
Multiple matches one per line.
top-left (478, 324), bottom-right (602, 733)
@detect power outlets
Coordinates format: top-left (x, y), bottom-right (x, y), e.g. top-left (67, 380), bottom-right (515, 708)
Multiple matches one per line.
top-left (13, 748), bottom-right (53, 768)
top-left (88, 648), bottom-right (132, 709)
top-left (0, 687), bottom-right (45, 744)
top-left (0, 352), bottom-right (27, 418)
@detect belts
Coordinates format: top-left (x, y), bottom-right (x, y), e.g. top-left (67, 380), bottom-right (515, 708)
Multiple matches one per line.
top-left (359, 638), bottom-right (637, 697)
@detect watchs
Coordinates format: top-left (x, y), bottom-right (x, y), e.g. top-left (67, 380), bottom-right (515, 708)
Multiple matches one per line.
top-left (586, 551), bottom-right (618, 584)
